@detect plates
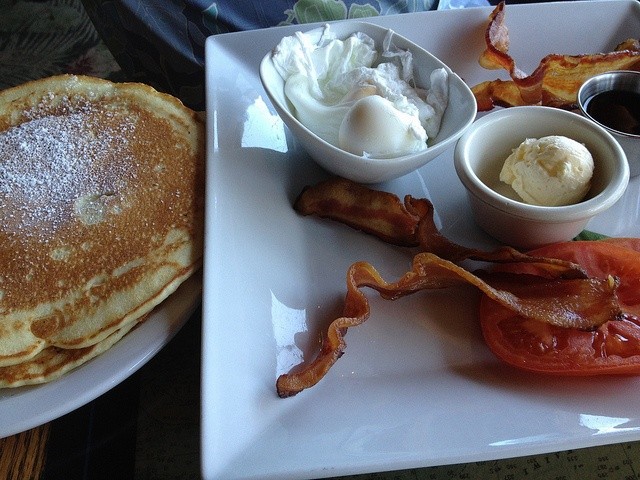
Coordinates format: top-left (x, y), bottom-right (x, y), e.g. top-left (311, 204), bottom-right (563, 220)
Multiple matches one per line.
top-left (199, 0), bottom-right (640, 480)
top-left (1, 273), bottom-right (203, 440)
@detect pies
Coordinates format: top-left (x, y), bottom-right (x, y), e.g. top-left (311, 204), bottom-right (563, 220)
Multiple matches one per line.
top-left (0, 73), bottom-right (209, 391)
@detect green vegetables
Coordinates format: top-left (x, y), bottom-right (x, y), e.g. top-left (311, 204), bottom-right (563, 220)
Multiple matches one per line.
top-left (572, 229), bottom-right (609, 241)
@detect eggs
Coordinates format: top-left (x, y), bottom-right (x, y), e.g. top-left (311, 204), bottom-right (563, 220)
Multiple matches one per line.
top-left (271, 21), bottom-right (448, 160)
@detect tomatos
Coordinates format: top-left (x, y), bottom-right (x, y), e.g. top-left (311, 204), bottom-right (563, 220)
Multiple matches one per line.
top-left (478, 237), bottom-right (639, 375)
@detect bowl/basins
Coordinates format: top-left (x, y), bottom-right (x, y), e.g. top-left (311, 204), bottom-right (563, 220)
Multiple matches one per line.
top-left (453, 105), bottom-right (631, 251)
top-left (577, 70), bottom-right (640, 178)
top-left (259, 21), bottom-right (478, 183)
